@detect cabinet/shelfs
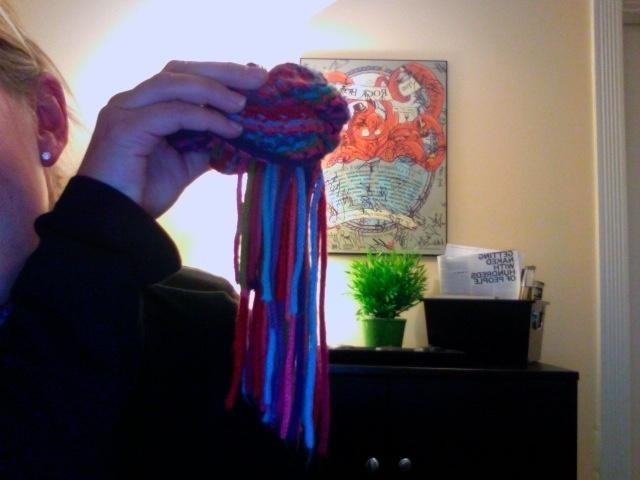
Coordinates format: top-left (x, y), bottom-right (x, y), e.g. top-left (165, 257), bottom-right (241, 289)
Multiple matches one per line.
top-left (317, 374), bottom-right (575, 480)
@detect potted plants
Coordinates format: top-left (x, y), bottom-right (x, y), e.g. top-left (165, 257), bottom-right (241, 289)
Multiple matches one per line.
top-left (341, 241), bottom-right (428, 349)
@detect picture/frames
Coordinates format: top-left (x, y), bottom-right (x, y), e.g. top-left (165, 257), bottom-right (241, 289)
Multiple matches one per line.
top-left (298, 57), bottom-right (449, 255)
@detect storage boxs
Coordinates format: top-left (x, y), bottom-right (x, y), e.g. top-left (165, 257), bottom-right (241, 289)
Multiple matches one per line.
top-left (421, 298), bottom-right (550, 364)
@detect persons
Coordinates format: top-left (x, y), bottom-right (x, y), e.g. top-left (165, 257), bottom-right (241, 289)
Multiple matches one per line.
top-left (0, 1), bottom-right (278, 480)
